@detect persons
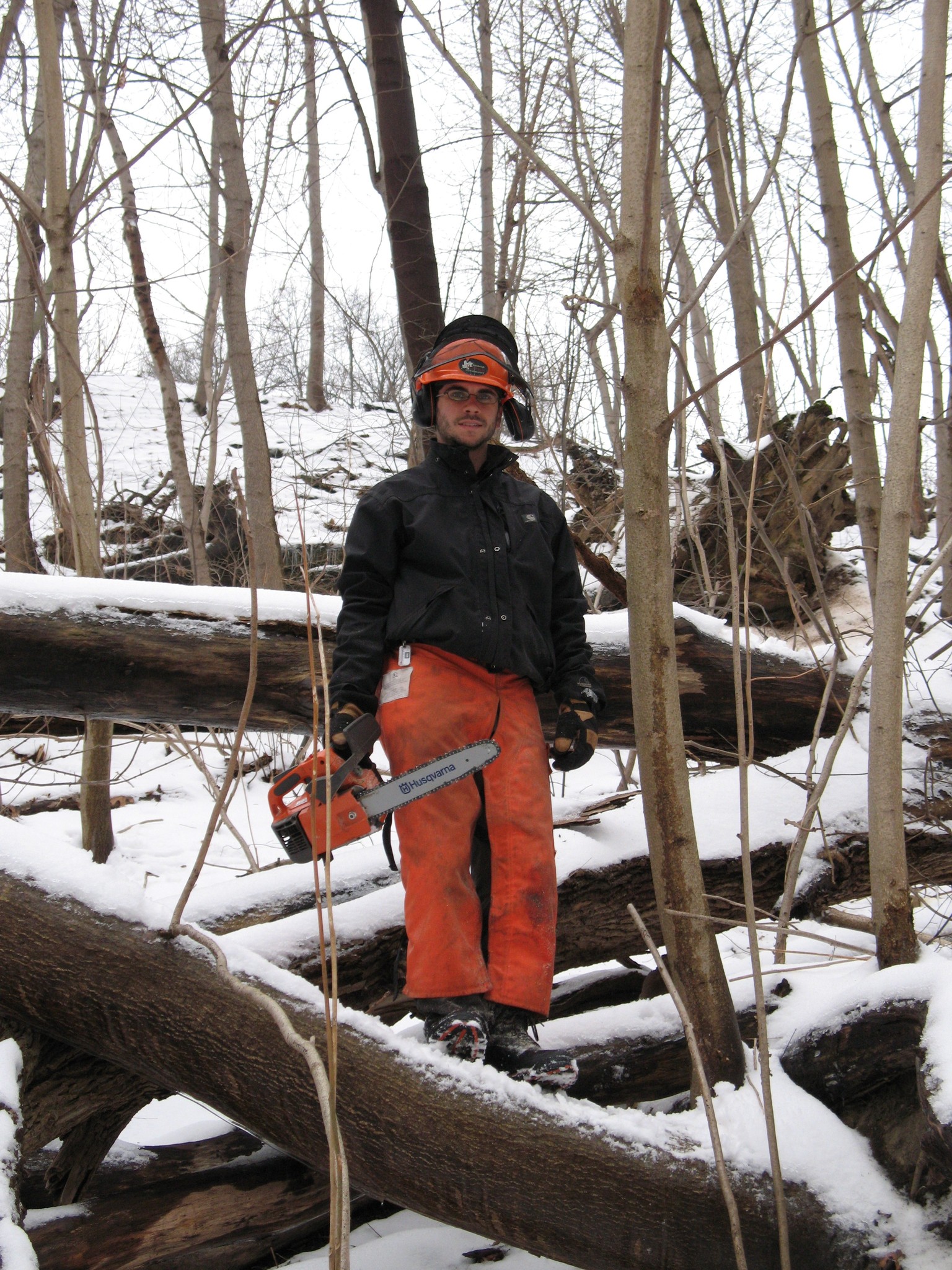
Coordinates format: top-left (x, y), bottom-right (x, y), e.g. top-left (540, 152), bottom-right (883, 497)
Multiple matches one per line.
top-left (325, 338), bottom-right (598, 1087)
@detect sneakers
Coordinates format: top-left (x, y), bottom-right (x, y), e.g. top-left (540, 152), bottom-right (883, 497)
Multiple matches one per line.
top-left (483, 1001), bottom-right (578, 1092)
top-left (424, 1009), bottom-right (488, 1063)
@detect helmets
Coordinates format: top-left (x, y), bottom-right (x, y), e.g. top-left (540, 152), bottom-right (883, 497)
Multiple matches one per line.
top-left (416, 315), bottom-right (518, 406)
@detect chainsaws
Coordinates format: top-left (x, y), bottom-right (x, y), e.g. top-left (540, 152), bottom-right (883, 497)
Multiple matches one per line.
top-left (268, 711), bottom-right (503, 864)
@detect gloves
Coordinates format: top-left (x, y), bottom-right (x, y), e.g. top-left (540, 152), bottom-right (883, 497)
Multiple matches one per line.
top-left (322, 690), bottom-right (378, 769)
top-left (549, 677), bottom-right (607, 772)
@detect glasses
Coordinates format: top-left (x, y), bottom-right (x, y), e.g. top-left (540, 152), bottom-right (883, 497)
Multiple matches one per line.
top-left (435, 389), bottom-right (501, 404)
